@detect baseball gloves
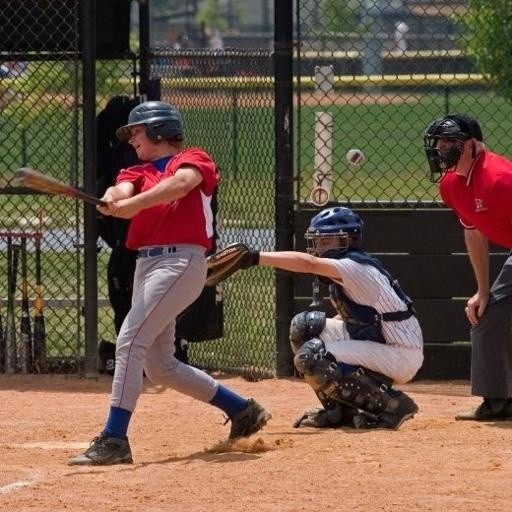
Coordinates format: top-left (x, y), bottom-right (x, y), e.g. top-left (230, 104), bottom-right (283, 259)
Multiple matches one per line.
top-left (205, 242), bottom-right (259, 287)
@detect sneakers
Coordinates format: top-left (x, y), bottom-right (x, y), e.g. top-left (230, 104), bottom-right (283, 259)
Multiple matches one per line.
top-left (221, 398), bottom-right (272, 444)
top-left (357, 391), bottom-right (419, 429)
top-left (67, 431), bottom-right (136, 465)
top-left (313, 406), bottom-right (359, 428)
top-left (454, 399), bottom-right (511, 421)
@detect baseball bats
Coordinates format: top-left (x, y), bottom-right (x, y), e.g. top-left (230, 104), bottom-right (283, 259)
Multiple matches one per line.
top-left (13, 168), bottom-right (108, 207)
top-left (0, 233), bottom-right (49, 373)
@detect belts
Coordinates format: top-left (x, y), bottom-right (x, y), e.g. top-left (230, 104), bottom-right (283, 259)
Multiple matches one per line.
top-left (137, 247), bottom-right (176, 260)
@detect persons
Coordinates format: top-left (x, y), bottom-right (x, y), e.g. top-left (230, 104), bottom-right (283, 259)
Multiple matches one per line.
top-left (423, 114), bottom-right (512, 420)
top-left (98, 94), bottom-right (138, 334)
top-left (68, 100), bottom-right (274, 464)
top-left (203, 207), bottom-right (424, 431)
top-left (174, 20), bottom-right (228, 78)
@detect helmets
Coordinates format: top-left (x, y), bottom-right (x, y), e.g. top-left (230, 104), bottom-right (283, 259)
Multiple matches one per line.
top-left (308, 207), bottom-right (366, 241)
top-left (115, 101), bottom-right (185, 145)
top-left (425, 114), bottom-right (482, 142)
